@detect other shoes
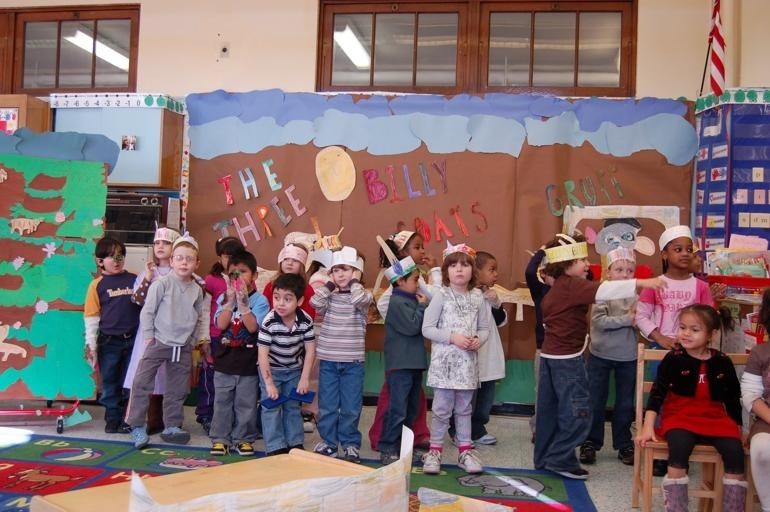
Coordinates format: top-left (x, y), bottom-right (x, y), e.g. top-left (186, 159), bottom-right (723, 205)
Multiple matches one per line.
top-left (302, 413), bottom-right (315, 432)
top-left (201, 419), bottom-right (214, 434)
top-left (106, 418), bottom-right (130, 434)
top-left (411, 450), bottom-right (423, 467)
top-left (267, 444), bottom-right (304, 457)
top-left (381, 450), bottom-right (398, 464)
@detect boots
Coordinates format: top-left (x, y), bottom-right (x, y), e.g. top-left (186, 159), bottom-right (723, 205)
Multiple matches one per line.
top-left (661, 474), bottom-right (688, 512)
top-left (722, 477), bottom-right (747, 512)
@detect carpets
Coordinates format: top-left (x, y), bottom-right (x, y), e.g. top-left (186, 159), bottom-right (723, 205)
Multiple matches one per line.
top-left (0, 435), bottom-right (601, 511)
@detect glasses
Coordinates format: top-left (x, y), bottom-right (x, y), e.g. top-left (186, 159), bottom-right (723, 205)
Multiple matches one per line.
top-left (173, 254), bottom-right (196, 262)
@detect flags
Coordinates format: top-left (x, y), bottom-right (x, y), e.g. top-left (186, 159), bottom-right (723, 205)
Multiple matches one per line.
top-left (707, 2), bottom-right (727, 98)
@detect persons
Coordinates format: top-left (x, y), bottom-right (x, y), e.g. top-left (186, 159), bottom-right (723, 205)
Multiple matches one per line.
top-left (639, 303), bottom-right (747, 510)
top-left (84, 236), bottom-right (133, 433)
top-left (131, 232), bottom-right (209, 447)
top-left (369, 230), bottom-right (509, 474)
top-left (520, 231), bottom-right (668, 481)
top-left (202, 236), bottom-right (316, 454)
top-left (308, 246), bottom-right (373, 461)
top-left (635, 225), bottom-right (727, 475)
top-left (122, 221), bottom-right (182, 418)
top-left (741, 287), bottom-right (770, 511)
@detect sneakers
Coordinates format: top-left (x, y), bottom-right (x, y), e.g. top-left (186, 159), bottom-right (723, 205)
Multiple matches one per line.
top-left (532, 440), bottom-right (667, 479)
top-left (233, 440), bottom-right (255, 456)
top-left (160, 426), bottom-right (191, 445)
top-left (474, 434), bottom-right (495, 445)
top-left (313, 441), bottom-right (339, 459)
top-left (456, 450), bottom-right (482, 472)
top-left (450, 436), bottom-right (474, 449)
top-left (132, 423), bottom-right (149, 448)
top-left (343, 445), bottom-right (361, 464)
top-left (211, 443), bottom-right (231, 457)
top-left (421, 449), bottom-right (443, 473)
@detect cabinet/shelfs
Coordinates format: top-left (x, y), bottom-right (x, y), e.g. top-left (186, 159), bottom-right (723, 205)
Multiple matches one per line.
top-left (0, 93), bottom-right (50, 135)
top-left (106, 192), bottom-right (169, 278)
top-left (50, 93), bottom-right (184, 189)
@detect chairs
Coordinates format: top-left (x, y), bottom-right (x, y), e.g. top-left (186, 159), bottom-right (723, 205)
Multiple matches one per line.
top-left (632, 342), bottom-right (725, 512)
top-left (707, 352), bottom-right (763, 512)
